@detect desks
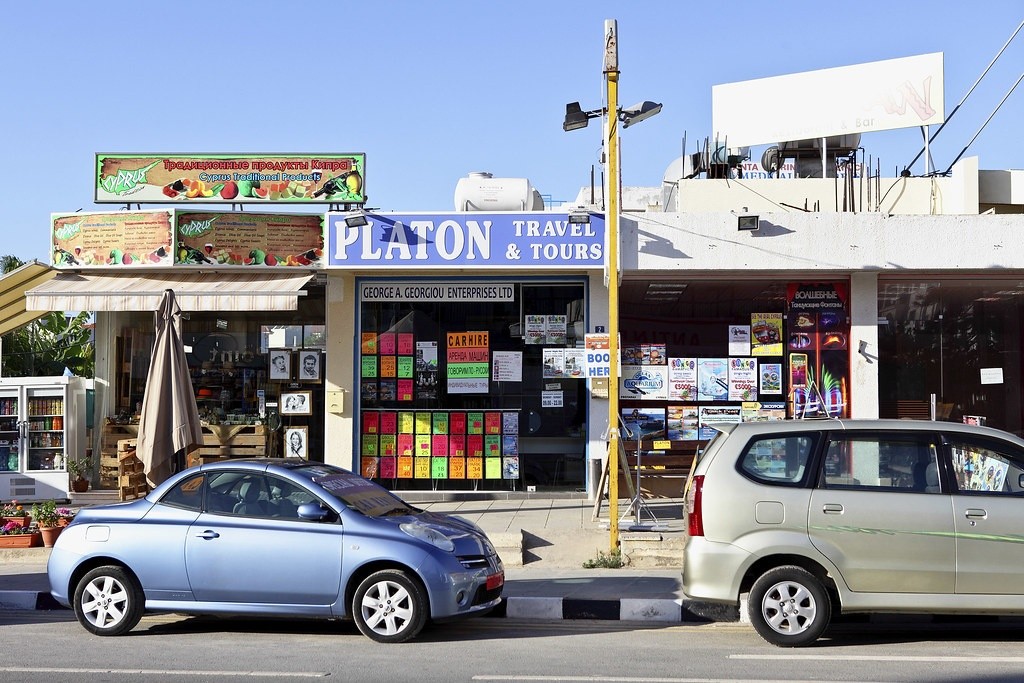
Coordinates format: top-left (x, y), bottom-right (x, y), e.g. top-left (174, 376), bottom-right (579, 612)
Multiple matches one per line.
top-left (99, 425), bottom-right (278, 491)
top-left (117, 437), bottom-right (200, 502)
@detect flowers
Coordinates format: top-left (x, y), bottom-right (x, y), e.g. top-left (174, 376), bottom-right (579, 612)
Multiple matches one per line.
top-left (0, 499), bottom-right (29, 517)
top-left (0, 521), bottom-right (40, 535)
top-left (55, 508), bottom-right (73, 518)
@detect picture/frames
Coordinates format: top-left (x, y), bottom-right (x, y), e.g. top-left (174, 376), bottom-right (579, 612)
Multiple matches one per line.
top-left (298, 348), bottom-right (323, 381)
top-left (278, 390), bottom-right (312, 416)
top-left (268, 348), bottom-right (292, 383)
top-left (283, 426), bottom-right (309, 461)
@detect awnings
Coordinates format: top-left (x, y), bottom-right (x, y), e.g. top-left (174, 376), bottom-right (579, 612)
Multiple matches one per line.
top-left (0, 260), bottom-right (61, 336)
top-left (24, 274), bottom-right (314, 311)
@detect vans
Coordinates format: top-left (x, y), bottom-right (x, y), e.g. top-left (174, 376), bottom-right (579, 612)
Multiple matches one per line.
top-left (679, 419), bottom-right (1024, 648)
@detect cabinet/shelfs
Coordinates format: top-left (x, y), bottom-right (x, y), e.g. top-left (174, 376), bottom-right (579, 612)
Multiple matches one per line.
top-left (192, 369), bottom-right (243, 409)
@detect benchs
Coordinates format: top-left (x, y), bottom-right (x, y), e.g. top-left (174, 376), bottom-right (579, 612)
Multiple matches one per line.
top-left (179, 495), bottom-right (239, 513)
top-left (606, 441), bottom-right (709, 515)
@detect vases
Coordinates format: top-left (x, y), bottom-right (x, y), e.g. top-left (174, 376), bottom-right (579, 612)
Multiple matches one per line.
top-left (56, 516), bottom-right (73, 527)
top-left (0, 533), bottom-right (41, 549)
top-left (0, 516), bottom-right (32, 528)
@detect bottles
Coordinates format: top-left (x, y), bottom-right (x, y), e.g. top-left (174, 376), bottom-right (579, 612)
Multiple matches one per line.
top-left (0, 398), bottom-right (64, 471)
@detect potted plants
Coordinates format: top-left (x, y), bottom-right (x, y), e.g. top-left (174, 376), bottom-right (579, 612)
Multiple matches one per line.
top-left (67, 457), bottom-right (95, 492)
top-left (32, 498), bottom-right (64, 548)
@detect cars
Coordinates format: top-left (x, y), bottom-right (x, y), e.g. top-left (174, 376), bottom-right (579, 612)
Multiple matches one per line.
top-left (46, 457), bottom-right (506, 644)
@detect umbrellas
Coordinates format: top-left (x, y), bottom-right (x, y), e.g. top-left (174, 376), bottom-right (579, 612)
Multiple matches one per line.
top-left (136, 288), bottom-right (203, 487)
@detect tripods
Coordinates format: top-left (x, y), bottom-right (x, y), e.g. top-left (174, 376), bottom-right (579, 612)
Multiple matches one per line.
top-left (617, 432), bottom-right (659, 527)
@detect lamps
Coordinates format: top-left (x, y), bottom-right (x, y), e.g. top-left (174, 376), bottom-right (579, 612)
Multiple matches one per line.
top-left (569, 210), bottom-right (590, 223)
top-left (738, 212), bottom-right (759, 231)
top-left (344, 210), bottom-right (368, 228)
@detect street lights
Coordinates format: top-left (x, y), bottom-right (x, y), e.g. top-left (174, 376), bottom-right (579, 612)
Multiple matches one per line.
top-left (564, 18), bottom-right (664, 548)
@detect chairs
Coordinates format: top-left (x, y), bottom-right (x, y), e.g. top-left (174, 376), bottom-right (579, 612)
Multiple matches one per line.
top-left (266, 483), bottom-right (298, 517)
top-left (233, 480), bottom-right (264, 515)
top-left (913, 461), bottom-right (940, 493)
